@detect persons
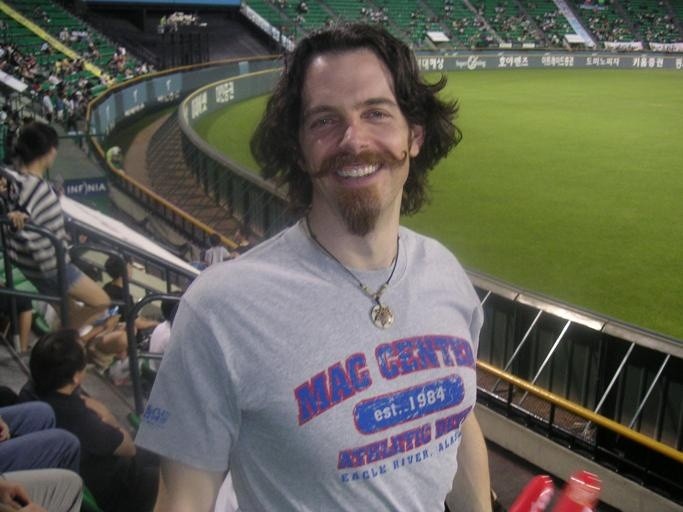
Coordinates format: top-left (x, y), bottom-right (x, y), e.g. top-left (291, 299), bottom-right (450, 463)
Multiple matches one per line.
top-left (135, 14), bottom-right (492, 512)
top-left (270, 0), bottom-right (682, 55)
top-left (223, 226), bottom-right (253, 260)
top-left (205, 233), bottom-right (228, 267)
top-left (0, 8), bottom-right (207, 512)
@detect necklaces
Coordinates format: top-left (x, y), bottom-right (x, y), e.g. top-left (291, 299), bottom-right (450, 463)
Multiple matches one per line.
top-left (304, 204), bottom-right (398, 328)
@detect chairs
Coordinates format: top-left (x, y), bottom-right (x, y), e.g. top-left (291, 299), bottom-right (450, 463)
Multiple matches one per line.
top-left (0, 250), bottom-right (159, 512)
